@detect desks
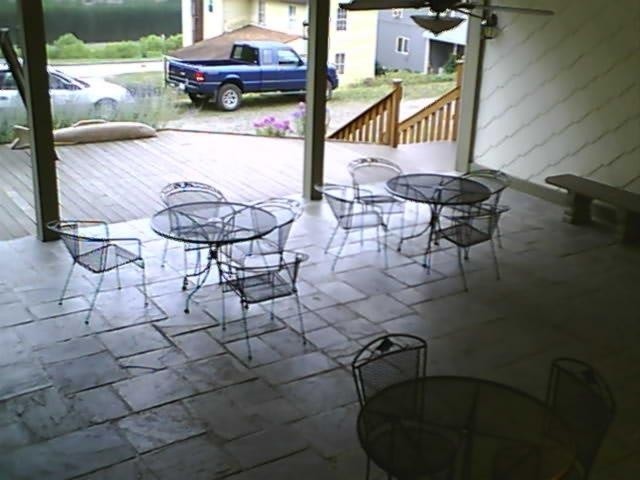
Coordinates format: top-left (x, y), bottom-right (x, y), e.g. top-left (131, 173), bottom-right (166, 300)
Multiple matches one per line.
top-left (384, 173), bottom-right (491, 267)
top-left (358, 376), bottom-right (576, 480)
top-left (150, 202), bottom-right (278, 315)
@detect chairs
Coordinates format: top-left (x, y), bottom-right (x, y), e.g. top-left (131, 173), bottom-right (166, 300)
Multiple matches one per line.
top-left (347, 157), bottom-right (409, 253)
top-left (216, 250), bottom-right (309, 360)
top-left (443, 169), bottom-right (512, 248)
top-left (544, 358), bottom-right (616, 479)
top-left (160, 181), bottom-right (236, 269)
top-left (314, 183), bottom-right (388, 271)
top-left (45, 219), bottom-right (148, 325)
top-left (223, 197), bottom-right (304, 250)
top-left (352, 334), bottom-right (427, 480)
top-left (426, 202), bottom-right (512, 291)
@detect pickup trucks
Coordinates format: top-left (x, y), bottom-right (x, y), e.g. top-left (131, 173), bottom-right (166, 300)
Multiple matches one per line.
top-left (159, 39), bottom-right (338, 114)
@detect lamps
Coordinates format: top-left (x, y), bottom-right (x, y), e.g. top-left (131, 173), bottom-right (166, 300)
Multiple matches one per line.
top-left (480, 14), bottom-right (498, 39)
top-left (410, 1), bottom-right (464, 35)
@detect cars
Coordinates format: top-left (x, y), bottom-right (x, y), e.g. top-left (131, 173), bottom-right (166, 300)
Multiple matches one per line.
top-left (0, 62), bottom-right (136, 123)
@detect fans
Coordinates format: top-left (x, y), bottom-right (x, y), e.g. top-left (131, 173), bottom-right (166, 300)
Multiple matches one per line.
top-left (338, 0), bottom-right (555, 16)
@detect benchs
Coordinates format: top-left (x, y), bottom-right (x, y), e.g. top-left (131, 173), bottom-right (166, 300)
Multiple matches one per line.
top-left (546, 173), bottom-right (640, 241)
